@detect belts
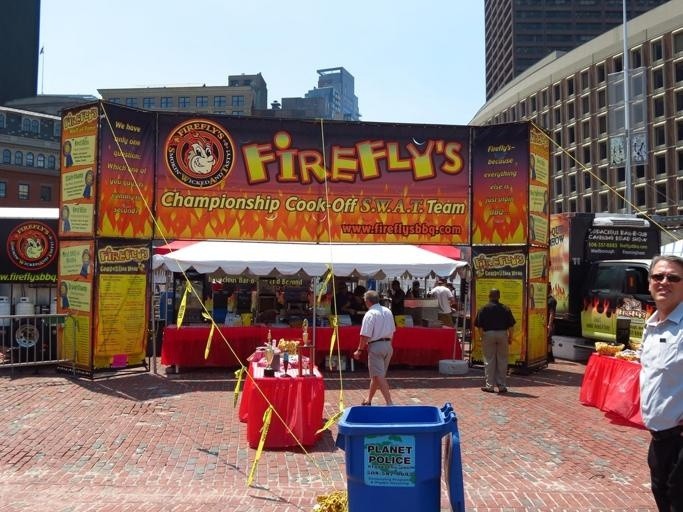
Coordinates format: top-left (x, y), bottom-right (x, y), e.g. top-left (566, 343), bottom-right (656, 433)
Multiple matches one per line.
top-left (648, 426), bottom-right (683, 440)
top-left (368, 338), bottom-right (391, 344)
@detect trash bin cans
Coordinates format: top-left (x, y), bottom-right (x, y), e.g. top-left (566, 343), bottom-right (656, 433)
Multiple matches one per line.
top-left (335, 401), bottom-right (464, 512)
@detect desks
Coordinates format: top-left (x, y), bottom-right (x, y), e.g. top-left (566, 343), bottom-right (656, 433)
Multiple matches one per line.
top-left (166, 325), bottom-right (457, 373)
top-left (590, 351), bottom-right (645, 427)
top-left (248, 349), bottom-right (324, 449)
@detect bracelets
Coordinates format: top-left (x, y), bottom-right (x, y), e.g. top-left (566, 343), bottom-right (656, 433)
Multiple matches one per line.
top-left (356, 348), bottom-right (363, 352)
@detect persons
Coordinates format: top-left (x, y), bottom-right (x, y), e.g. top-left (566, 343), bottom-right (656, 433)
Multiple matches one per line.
top-left (331, 277), bottom-right (456, 326)
top-left (635, 254), bottom-right (682, 512)
top-left (352, 291), bottom-right (398, 405)
top-left (473, 287), bottom-right (517, 393)
top-left (547, 282), bottom-right (557, 364)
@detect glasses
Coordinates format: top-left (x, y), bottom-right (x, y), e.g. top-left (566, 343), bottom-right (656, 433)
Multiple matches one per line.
top-left (649, 274), bottom-right (682, 282)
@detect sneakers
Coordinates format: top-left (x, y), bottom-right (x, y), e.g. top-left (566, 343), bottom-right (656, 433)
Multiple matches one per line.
top-left (481, 387), bottom-right (494, 393)
top-left (499, 388), bottom-right (507, 393)
top-left (361, 400), bottom-right (371, 405)
